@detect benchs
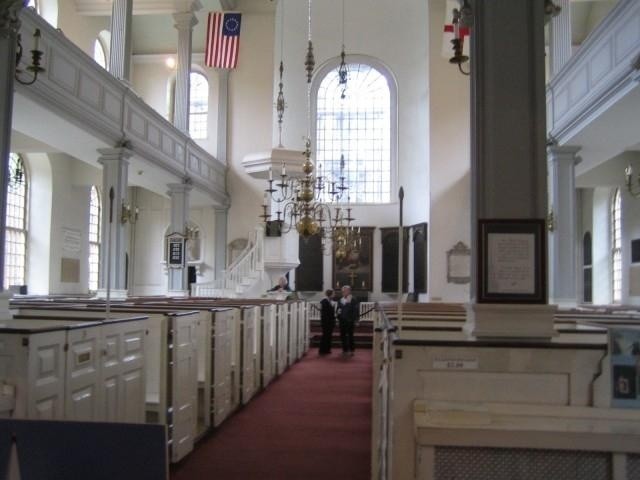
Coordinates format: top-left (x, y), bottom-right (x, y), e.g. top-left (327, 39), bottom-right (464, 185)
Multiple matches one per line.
top-left (369, 300), bottom-right (640, 480)
top-left (0, 292), bottom-right (315, 465)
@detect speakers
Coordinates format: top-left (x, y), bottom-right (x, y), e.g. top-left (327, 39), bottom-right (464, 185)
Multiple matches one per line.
top-left (266, 221), bottom-right (281, 236)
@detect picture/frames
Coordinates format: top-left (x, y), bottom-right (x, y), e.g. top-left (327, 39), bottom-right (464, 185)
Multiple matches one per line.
top-left (330, 223), bottom-right (377, 294)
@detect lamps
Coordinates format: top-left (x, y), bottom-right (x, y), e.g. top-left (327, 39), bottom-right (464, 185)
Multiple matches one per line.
top-left (121, 199), bottom-right (141, 225)
top-left (14, 28), bottom-right (44, 91)
top-left (258, 0), bottom-right (363, 262)
top-left (185, 224), bottom-right (200, 242)
top-left (7, 155), bottom-right (25, 192)
top-left (623, 163), bottom-right (639, 199)
top-left (448, 6), bottom-right (470, 78)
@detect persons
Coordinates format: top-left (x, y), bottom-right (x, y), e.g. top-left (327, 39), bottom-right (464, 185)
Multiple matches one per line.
top-left (336, 285), bottom-right (359, 355)
top-left (271, 277), bottom-right (292, 292)
top-left (320, 290), bottom-right (335, 354)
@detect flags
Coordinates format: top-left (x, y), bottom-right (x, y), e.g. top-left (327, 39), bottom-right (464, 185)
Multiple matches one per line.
top-left (204, 13), bottom-right (241, 70)
